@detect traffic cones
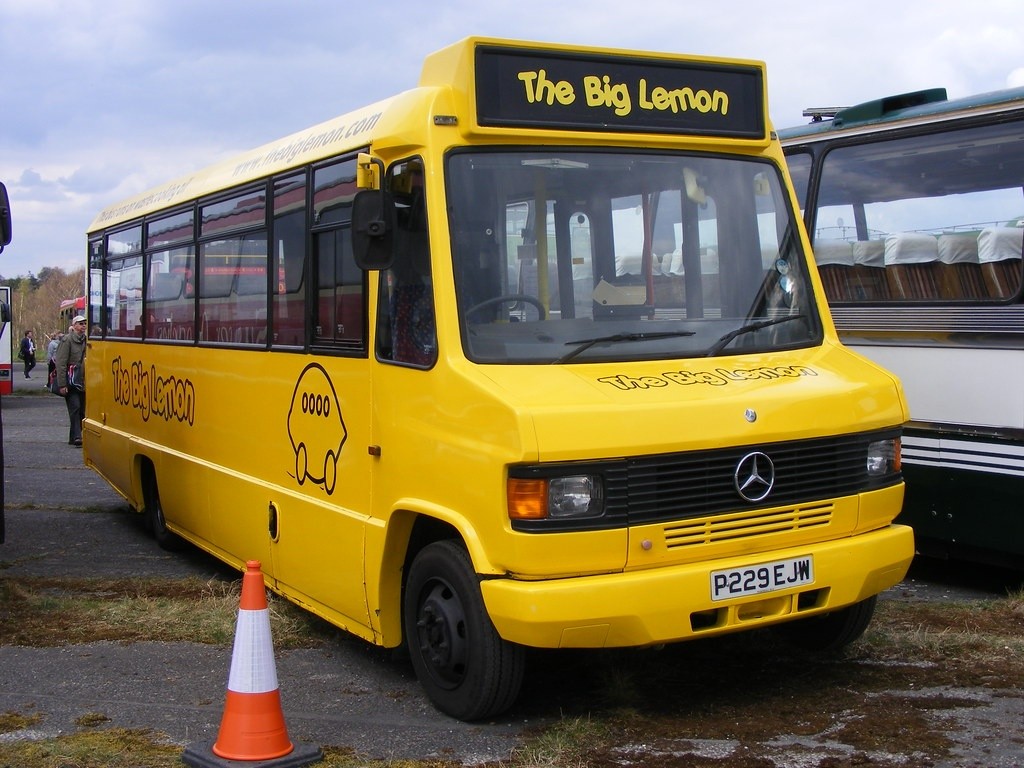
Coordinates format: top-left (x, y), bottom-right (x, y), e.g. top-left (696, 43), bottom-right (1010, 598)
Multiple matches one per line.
top-left (177, 560), bottom-right (326, 768)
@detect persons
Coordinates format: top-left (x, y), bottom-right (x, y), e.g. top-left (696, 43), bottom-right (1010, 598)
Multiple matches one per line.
top-left (44, 325), bottom-right (75, 394)
top-left (21, 330), bottom-right (37, 380)
top-left (56, 315), bottom-right (87, 448)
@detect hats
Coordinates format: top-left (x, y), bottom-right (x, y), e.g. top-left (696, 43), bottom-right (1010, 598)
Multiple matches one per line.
top-left (72, 316), bottom-right (86, 325)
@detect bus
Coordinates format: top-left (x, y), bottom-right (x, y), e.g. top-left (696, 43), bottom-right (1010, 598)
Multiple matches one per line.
top-left (110, 245), bottom-right (288, 346)
top-left (503, 84), bottom-right (1024, 584)
top-left (0, 284), bottom-right (14, 397)
top-left (74, 36), bottom-right (915, 726)
top-left (59, 296), bottom-right (89, 338)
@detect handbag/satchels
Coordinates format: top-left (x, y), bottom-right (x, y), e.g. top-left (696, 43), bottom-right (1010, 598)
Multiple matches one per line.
top-left (18, 352), bottom-right (25, 360)
top-left (50, 356), bottom-right (85, 397)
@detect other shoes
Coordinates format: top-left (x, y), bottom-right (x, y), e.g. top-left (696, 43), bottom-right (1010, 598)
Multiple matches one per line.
top-left (24, 375), bottom-right (32, 380)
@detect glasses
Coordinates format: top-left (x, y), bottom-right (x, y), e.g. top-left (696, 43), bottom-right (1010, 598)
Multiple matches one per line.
top-left (78, 322), bottom-right (87, 325)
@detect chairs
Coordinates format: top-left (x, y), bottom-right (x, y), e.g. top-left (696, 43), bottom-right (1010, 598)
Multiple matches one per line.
top-left (569, 227), bottom-right (1024, 307)
